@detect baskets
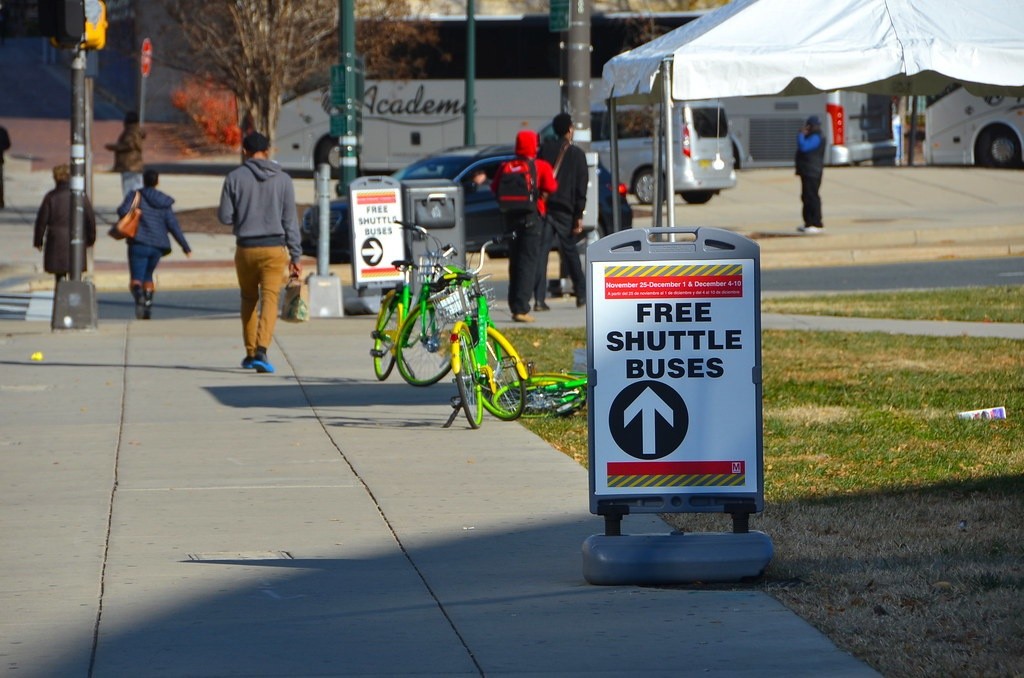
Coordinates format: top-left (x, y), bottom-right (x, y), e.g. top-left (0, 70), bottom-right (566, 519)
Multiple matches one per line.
top-left (433, 272), bottom-right (496, 325)
top-left (416, 254), bottom-right (461, 286)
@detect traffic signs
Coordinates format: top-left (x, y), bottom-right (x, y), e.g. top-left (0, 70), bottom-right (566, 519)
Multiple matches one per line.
top-left (580, 225), bottom-right (763, 515)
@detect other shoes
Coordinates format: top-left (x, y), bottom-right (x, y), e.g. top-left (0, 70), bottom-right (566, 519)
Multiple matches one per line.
top-left (535, 300), bottom-right (548, 311)
top-left (798, 224), bottom-right (822, 235)
top-left (513, 313), bottom-right (535, 323)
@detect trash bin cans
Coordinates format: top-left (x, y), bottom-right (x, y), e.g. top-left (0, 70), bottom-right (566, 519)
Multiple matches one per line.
top-left (401, 179), bottom-right (465, 295)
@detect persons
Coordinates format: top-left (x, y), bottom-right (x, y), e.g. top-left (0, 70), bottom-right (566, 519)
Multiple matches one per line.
top-left (472, 113), bottom-right (587, 322)
top-left (33, 164), bottom-right (96, 282)
top-left (216, 131), bottom-right (303, 373)
top-left (116, 169), bottom-right (191, 308)
top-left (794, 115), bottom-right (827, 234)
top-left (103, 110), bottom-right (146, 198)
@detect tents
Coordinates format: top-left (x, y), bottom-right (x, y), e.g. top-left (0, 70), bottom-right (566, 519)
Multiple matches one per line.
top-left (601, 0), bottom-right (1024, 242)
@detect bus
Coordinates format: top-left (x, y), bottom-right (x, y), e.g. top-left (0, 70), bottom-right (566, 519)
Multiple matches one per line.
top-left (268, 3), bottom-right (897, 180)
top-left (920, 85), bottom-right (1024, 167)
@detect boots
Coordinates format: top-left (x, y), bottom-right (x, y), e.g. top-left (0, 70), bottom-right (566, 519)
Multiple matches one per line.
top-left (134, 286), bottom-right (152, 318)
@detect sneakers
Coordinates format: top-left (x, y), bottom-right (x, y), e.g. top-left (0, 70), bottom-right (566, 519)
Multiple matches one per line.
top-left (242, 356), bottom-right (255, 369)
top-left (253, 351), bottom-right (274, 374)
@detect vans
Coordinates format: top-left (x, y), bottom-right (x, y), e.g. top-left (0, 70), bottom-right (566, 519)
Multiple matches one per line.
top-left (536, 103), bottom-right (733, 204)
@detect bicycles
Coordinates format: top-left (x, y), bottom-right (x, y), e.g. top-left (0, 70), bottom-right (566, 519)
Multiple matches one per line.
top-left (491, 367), bottom-right (588, 420)
top-left (369, 219), bottom-right (530, 430)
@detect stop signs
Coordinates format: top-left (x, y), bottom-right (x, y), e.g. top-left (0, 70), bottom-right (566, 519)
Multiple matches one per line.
top-left (141, 38), bottom-right (151, 76)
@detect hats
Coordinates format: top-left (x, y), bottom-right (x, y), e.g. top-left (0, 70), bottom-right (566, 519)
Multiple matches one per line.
top-left (807, 115), bottom-right (821, 126)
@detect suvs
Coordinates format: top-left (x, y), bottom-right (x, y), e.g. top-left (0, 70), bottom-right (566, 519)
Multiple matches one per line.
top-left (297, 144), bottom-right (632, 264)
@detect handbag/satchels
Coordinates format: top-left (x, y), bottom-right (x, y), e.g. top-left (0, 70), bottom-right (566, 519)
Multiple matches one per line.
top-left (281, 278), bottom-right (309, 321)
top-left (109, 191), bottom-right (144, 241)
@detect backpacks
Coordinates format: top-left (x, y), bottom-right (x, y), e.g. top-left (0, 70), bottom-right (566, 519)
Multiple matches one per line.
top-left (498, 158), bottom-right (537, 212)
